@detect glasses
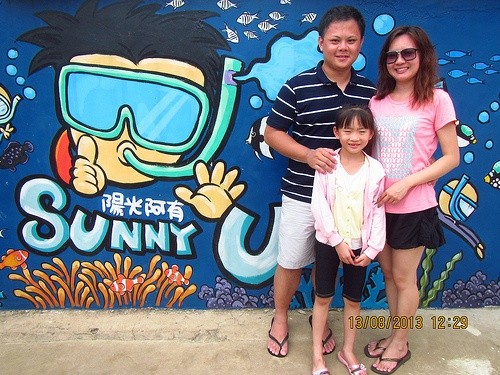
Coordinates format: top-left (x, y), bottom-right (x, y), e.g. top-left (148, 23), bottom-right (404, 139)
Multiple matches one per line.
top-left (384, 48), bottom-right (420, 64)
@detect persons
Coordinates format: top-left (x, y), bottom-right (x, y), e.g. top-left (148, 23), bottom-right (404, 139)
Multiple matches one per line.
top-left (365, 23), bottom-right (460, 373)
top-left (310, 104), bottom-right (387, 375)
top-left (264, 5), bottom-right (378, 358)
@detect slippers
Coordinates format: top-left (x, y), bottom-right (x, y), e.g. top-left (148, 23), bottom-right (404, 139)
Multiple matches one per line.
top-left (309, 314), bottom-right (336, 355)
top-left (371, 347), bottom-right (411, 375)
top-left (312, 359), bottom-right (330, 375)
top-left (267, 316), bottom-right (289, 358)
top-left (364, 333), bottom-right (409, 358)
top-left (338, 350), bottom-right (367, 375)
